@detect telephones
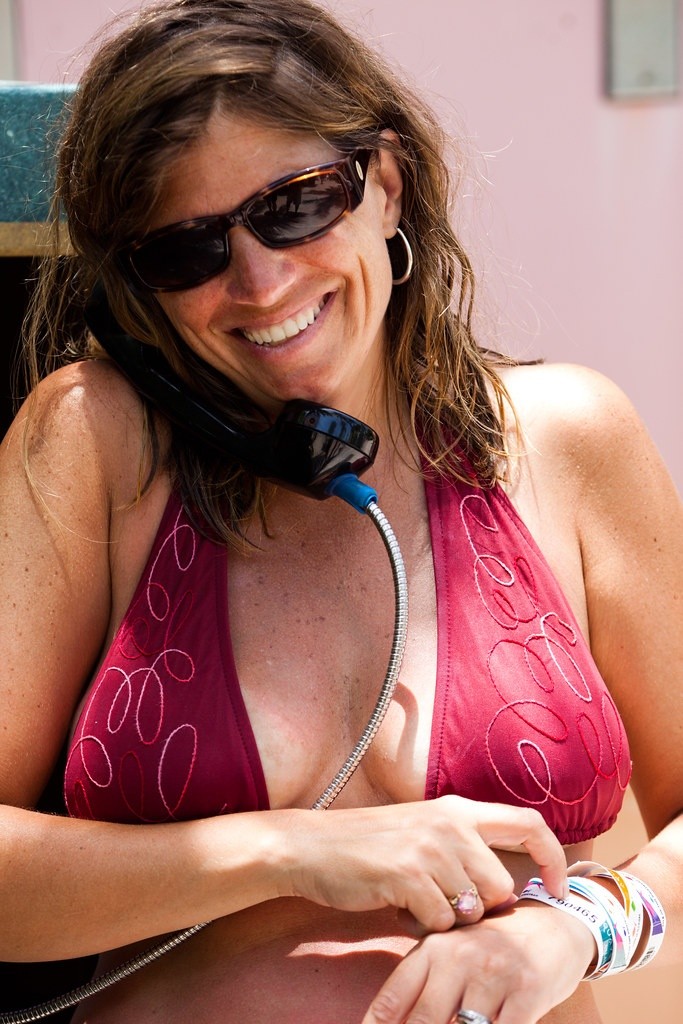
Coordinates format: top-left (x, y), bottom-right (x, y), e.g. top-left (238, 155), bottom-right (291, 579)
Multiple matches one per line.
top-left (51, 257), bottom-right (380, 502)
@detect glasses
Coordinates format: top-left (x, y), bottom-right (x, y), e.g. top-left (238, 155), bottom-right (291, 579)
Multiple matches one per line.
top-left (113, 122), bottom-right (394, 293)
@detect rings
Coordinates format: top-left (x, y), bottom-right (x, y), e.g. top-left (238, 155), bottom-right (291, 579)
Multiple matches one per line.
top-left (450, 882), bottom-right (478, 915)
top-left (457, 1009), bottom-right (493, 1024)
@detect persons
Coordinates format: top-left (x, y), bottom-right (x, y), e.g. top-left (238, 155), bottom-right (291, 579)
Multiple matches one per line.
top-left (263, 180), bottom-right (302, 214)
top-left (0, 0), bottom-right (683, 1024)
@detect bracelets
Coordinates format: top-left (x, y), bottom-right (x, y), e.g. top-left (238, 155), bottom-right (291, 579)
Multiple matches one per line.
top-left (519, 861), bottom-right (666, 980)
